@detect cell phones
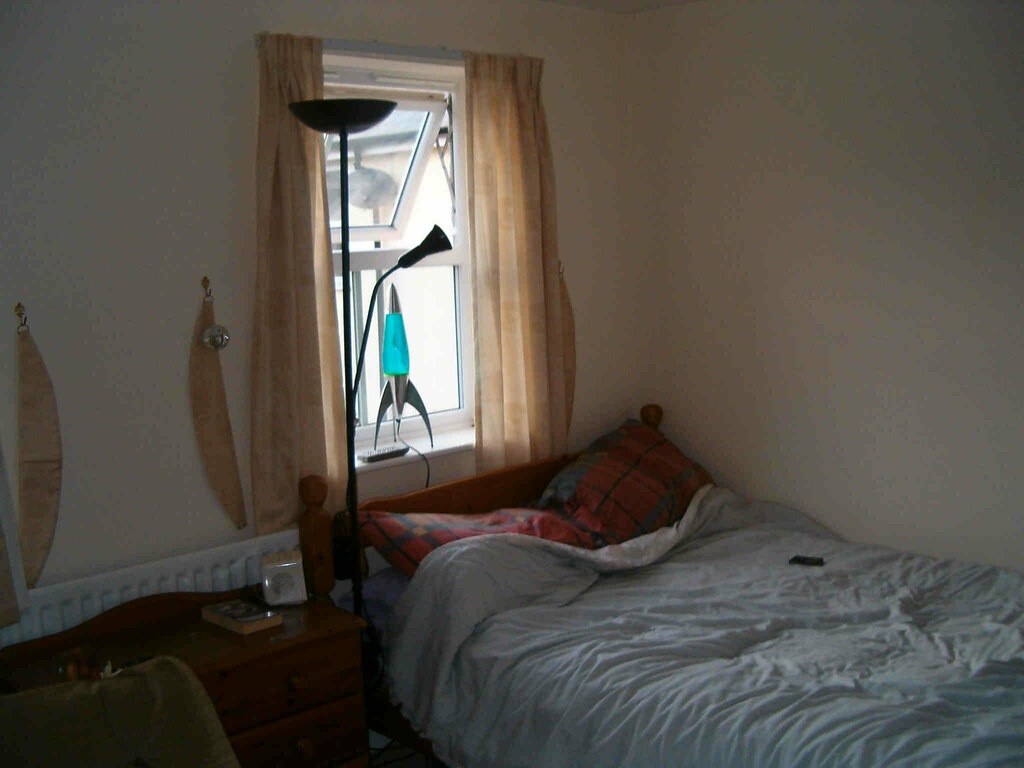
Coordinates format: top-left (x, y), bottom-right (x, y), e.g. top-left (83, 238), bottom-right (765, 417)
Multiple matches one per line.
top-left (789, 556), bottom-right (823, 566)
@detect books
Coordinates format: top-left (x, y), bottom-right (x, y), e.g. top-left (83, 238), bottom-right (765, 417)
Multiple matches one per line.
top-left (259, 550), bottom-right (309, 606)
top-left (201, 597), bottom-right (284, 636)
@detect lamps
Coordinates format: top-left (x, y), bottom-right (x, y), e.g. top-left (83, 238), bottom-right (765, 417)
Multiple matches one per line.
top-left (291, 98), bottom-right (453, 608)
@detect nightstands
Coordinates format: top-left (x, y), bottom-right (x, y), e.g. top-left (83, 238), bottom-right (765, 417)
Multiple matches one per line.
top-left (0, 583), bottom-right (370, 768)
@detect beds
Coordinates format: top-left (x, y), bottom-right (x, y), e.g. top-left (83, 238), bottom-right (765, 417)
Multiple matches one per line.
top-left (296, 403), bottom-right (1024, 768)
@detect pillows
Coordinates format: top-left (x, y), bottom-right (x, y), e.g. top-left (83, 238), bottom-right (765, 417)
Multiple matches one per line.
top-left (346, 510), bottom-right (597, 585)
top-left (535, 418), bottom-right (715, 544)
